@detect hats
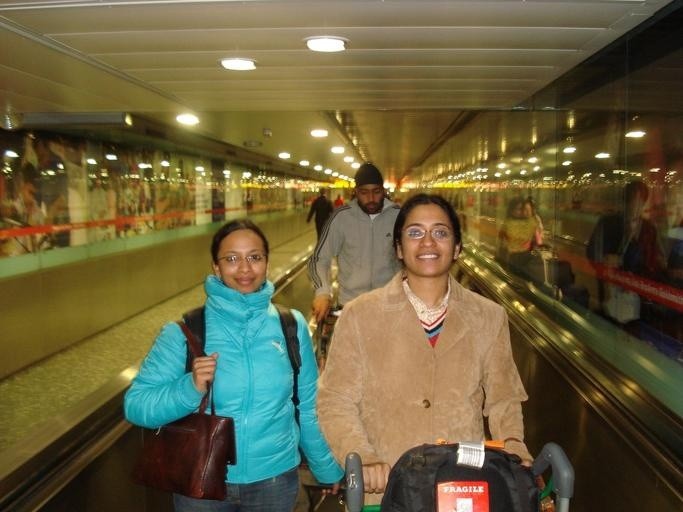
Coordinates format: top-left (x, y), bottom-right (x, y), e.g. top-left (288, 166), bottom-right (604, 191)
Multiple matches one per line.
top-left (354, 163), bottom-right (382, 187)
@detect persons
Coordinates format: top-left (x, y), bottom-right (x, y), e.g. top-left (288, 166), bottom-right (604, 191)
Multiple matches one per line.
top-left (313, 192), bottom-right (540, 511)
top-left (121, 217), bottom-right (345, 512)
top-left (499, 196), bottom-right (539, 278)
top-left (304, 160), bottom-right (404, 329)
top-left (66, 137), bottom-right (195, 244)
top-left (20, 136), bottom-right (70, 247)
top-left (305, 188), bottom-right (335, 240)
top-left (522, 198), bottom-right (544, 255)
top-left (2, 163), bottom-right (51, 257)
top-left (333, 194), bottom-right (345, 209)
top-left (584, 178), bottom-right (669, 341)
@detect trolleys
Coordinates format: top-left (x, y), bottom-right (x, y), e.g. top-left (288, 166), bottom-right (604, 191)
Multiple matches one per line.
top-left (314, 300), bottom-right (344, 369)
top-left (336, 438), bottom-right (578, 512)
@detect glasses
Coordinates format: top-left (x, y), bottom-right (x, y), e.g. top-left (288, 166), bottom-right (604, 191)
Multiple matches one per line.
top-left (215, 254), bottom-right (264, 265)
top-left (397, 228), bottom-right (453, 241)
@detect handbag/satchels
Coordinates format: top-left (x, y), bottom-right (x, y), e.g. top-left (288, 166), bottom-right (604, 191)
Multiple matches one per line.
top-left (381, 443), bottom-right (539, 511)
top-left (132, 415), bottom-right (236, 499)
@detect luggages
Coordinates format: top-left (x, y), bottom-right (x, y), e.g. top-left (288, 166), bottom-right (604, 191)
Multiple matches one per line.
top-left (301, 478), bottom-right (348, 512)
